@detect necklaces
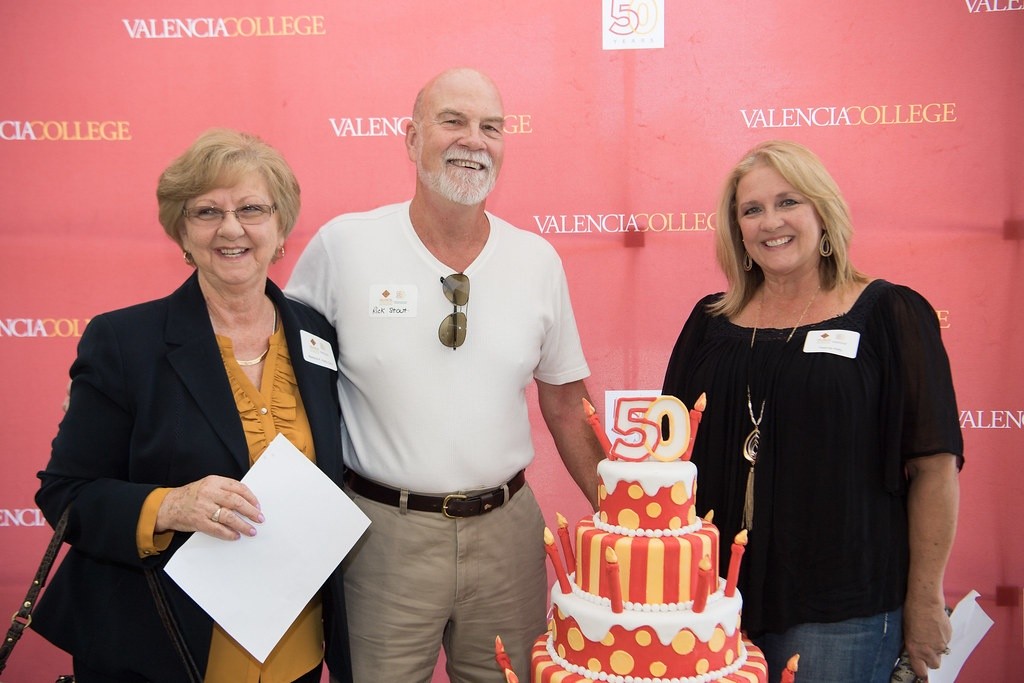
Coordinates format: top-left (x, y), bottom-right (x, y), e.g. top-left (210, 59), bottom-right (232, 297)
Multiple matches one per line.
top-left (743, 280), bottom-right (820, 534)
top-left (236, 303), bottom-right (277, 365)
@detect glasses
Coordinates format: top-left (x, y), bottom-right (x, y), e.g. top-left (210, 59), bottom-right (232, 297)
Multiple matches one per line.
top-left (438, 272), bottom-right (470, 351)
top-left (182, 203), bottom-right (277, 226)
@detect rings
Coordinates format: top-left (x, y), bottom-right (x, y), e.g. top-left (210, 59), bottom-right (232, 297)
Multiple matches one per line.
top-left (212, 507), bottom-right (223, 522)
top-left (944, 648), bottom-right (951, 655)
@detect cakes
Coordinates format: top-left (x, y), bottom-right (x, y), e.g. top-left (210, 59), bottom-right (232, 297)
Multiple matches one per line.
top-left (492, 388), bottom-right (801, 683)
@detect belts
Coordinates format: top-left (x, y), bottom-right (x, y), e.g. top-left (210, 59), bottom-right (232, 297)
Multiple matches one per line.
top-left (340, 463), bottom-right (527, 519)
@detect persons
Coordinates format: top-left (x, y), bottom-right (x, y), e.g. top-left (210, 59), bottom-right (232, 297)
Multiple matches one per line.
top-left (660, 139), bottom-right (964, 683)
top-left (36, 129), bottom-right (353, 683)
top-left (282, 67), bottom-right (610, 683)
